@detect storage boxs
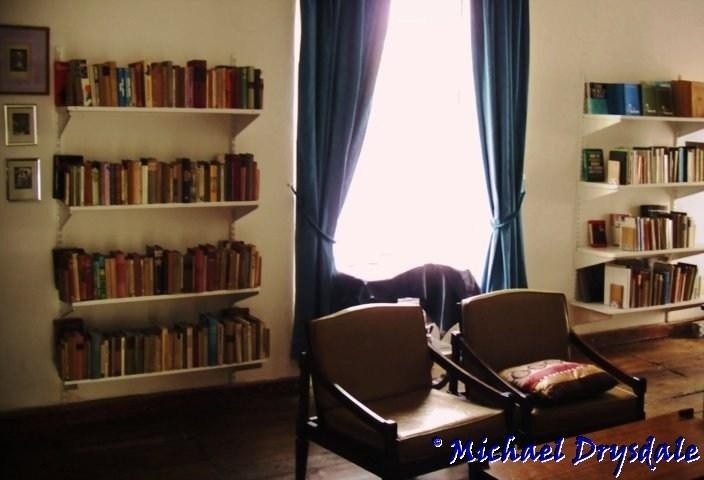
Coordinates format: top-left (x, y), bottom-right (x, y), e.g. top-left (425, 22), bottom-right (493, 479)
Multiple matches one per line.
top-left (672, 80), bottom-right (704, 117)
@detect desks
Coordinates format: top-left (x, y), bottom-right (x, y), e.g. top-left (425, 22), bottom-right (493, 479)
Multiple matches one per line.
top-left (468, 407), bottom-right (704, 480)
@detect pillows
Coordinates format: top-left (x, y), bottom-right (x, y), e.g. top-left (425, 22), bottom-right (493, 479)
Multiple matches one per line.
top-left (499, 359), bottom-right (619, 410)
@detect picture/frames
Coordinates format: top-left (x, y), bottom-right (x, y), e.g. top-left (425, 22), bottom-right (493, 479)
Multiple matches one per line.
top-left (4, 104), bottom-right (38, 146)
top-left (6, 158), bottom-right (41, 202)
top-left (0, 24), bottom-right (50, 95)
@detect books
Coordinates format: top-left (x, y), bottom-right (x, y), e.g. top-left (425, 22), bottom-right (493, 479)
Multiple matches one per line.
top-left (579, 79), bottom-right (703, 309)
top-left (52, 59), bottom-right (269, 381)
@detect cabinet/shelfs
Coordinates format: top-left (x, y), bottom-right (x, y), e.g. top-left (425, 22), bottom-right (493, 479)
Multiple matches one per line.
top-left (54, 46), bottom-right (268, 391)
top-left (570, 74), bottom-right (704, 322)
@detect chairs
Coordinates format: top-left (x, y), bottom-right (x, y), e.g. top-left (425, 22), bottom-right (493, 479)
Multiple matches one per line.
top-left (449, 288), bottom-right (646, 448)
top-left (295, 303), bottom-right (516, 480)
